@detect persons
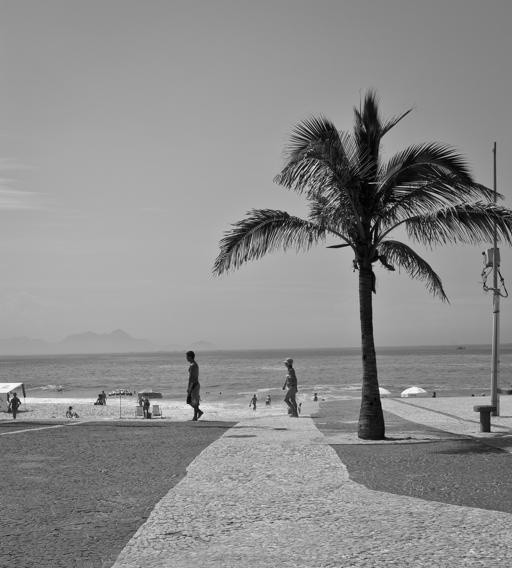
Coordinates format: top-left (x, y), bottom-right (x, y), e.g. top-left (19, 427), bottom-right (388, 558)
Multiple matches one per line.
top-left (283, 356), bottom-right (300, 416)
top-left (66, 405), bottom-right (80, 418)
top-left (139, 396), bottom-right (150, 417)
top-left (431, 392), bottom-right (436, 397)
top-left (8, 391), bottom-right (22, 420)
top-left (249, 394), bottom-right (258, 410)
top-left (185, 351), bottom-right (205, 420)
top-left (266, 394), bottom-right (271, 406)
top-left (101, 390), bottom-right (108, 406)
top-left (94, 393), bottom-right (103, 405)
top-left (313, 392), bottom-right (318, 401)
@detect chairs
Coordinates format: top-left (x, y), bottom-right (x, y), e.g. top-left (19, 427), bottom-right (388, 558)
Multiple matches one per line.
top-left (134, 406), bottom-right (145, 419)
top-left (152, 405), bottom-right (162, 418)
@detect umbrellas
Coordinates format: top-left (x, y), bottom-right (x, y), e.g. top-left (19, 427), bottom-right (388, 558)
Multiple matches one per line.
top-left (400, 385), bottom-right (426, 398)
top-left (109, 387), bottom-right (133, 418)
top-left (138, 387), bottom-right (163, 401)
top-left (378, 386), bottom-right (392, 397)
